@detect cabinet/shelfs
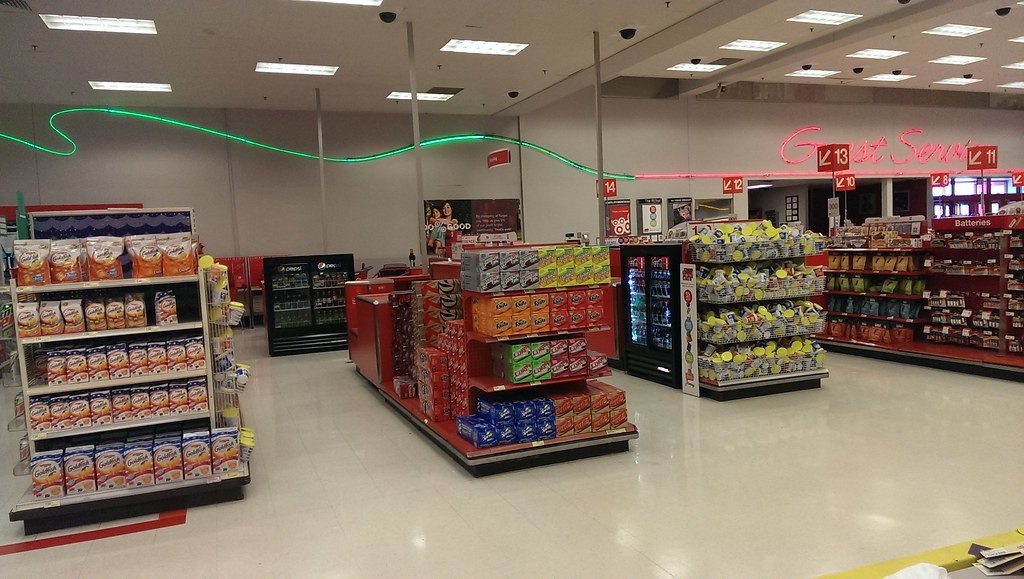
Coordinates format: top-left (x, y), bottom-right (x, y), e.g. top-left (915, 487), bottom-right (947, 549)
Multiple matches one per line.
top-left (0, 206), bottom-right (251, 535)
top-left (689, 235), bottom-right (829, 385)
top-left (821, 214), bottom-right (1024, 365)
top-left (464, 244), bottom-right (611, 391)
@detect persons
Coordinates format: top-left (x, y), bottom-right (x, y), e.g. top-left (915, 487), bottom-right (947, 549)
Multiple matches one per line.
top-left (428, 201), bottom-right (460, 254)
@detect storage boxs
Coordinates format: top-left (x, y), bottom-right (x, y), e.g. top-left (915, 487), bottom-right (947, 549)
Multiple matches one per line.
top-left (12, 288), bottom-right (241, 500)
top-left (386, 244), bottom-right (629, 449)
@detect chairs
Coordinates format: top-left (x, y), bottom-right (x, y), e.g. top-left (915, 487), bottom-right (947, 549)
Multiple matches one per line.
top-left (248, 255), bottom-right (265, 329)
top-left (215, 258), bottom-right (252, 329)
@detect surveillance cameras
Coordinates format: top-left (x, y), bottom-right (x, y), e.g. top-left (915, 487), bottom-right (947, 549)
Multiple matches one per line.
top-left (508, 92), bottom-right (519, 98)
top-left (378, 12), bottom-right (396, 23)
top-left (619, 28), bottom-right (636, 39)
top-left (802, 65), bottom-right (812, 70)
top-left (964, 74), bottom-right (973, 79)
top-left (995, 7), bottom-right (1011, 16)
top-left (691, 59), bottom-right (701, 64)
top-left (898, 0), bottom-right (911, 4)
top-left (892, 70), bottom-right (902, 75)
top-left (853, 68), bottom-right (863, 74)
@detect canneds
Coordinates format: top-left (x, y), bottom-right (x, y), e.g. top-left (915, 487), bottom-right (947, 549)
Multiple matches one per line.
top-left (629, 268), bottom-right (671, 348)
top-left (393, 376), bottom-right (416, 399)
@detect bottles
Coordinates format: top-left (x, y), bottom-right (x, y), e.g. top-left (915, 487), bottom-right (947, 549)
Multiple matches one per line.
top-left (629, 271), bottom-right (645, 293)
top-left (272, 271), bottom-right (310, 287)
top-left (651, 326), bottom-right (672, 349)
top-left (652, 301), bottom-right (670, 324)
top-left (316, 309), bottom-right (347, 326)
top-left (631, 297), bottom-right (646, 320)
top-left (409, 249), bottom-right (416, 267)
top-left (631, 323), bottom-right (646, 344)
top-left (315, 289), bottom-right (345, 307)
top-left (650, 270), bottom-right (670, 296)
top-left (273, 293), bottom-right (312, 310)
top-left (275, 310), bottom-right (312, 328)
top-left (313, 271), bottom-right (349, 287)
